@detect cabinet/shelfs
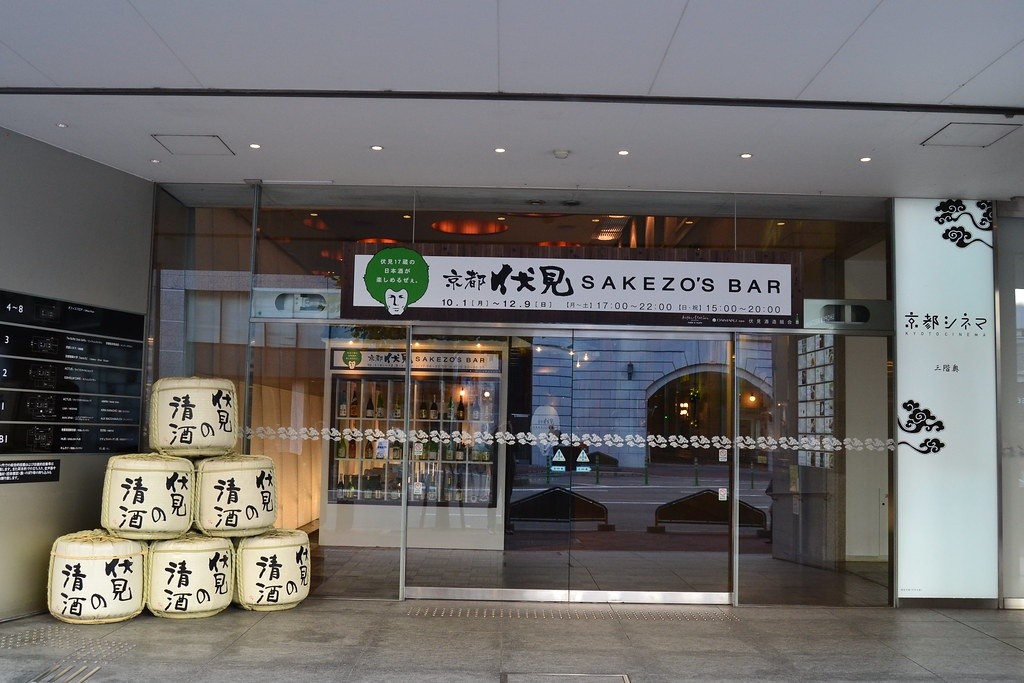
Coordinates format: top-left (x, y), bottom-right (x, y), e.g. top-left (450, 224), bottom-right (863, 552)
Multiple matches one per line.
top-left (328, 373), bottom-right (500, 507)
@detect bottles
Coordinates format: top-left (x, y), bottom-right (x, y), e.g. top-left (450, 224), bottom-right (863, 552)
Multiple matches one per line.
top-left (376, 393), bottom-right (384, 418)
top-left (471, 394), bottom-right (480, 420)
top-left (350, 390), bottom-right (358, 417)
top-left (336, 471), bottom-right (401, 501)
top-left (483, 396), bottom-right (496, 421)
top-left (446, 394), bottom-right (455, 421)
top-left (392, 432), bottom-right (400, 460)
top-left (429, 394), bottom-right (438, 420)
top-left (348, 431), bottom-right (357, 458)
top-left (393, 391), bottom-right (402, 419)
top-left (376, 432), bottom-right (384, 458)
top-left (365, 433), bottom-right (373, 459)
top-left (418, 393), bottom-right (427, 419)
top-left (339, 390), bottom-right (347, 417)
top-left (457, 395), bottom-right (466, 420)
top-left (411, 434), bottom-right (492, 461)
top-left (335, 432), bottom-right (346, 458)
top-left (366, 393), bottom-right (374, 418)
top-left (408, 473), bottom-right (493, 503)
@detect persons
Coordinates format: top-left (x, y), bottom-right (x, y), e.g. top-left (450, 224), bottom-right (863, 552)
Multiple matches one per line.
top-left (530, 394), bottom-right (562, 486)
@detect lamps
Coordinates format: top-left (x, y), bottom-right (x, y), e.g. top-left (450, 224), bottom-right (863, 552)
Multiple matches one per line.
top-left (749, 393), bottom-right (756, 402)
top-left (583, 352), bottom-right (589, 360)
top-left (626, 360), bottom-right (633, 381)
top-left (569, 349), bottom-right (574, 356)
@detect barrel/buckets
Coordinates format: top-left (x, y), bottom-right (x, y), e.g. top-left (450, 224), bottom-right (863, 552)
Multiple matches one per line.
top-left (231, 529), bottom-right (311, 612)
top-left (148, 537), bottom-right (231, 619)
top-left (100, 454), bottom-right (194, 540)
top-left (47, 532), bottom-right (146, 622)
top-left (149, 377), bottom-right (238, 457)
top-left (198, 456), bottom-right (278, 536)
